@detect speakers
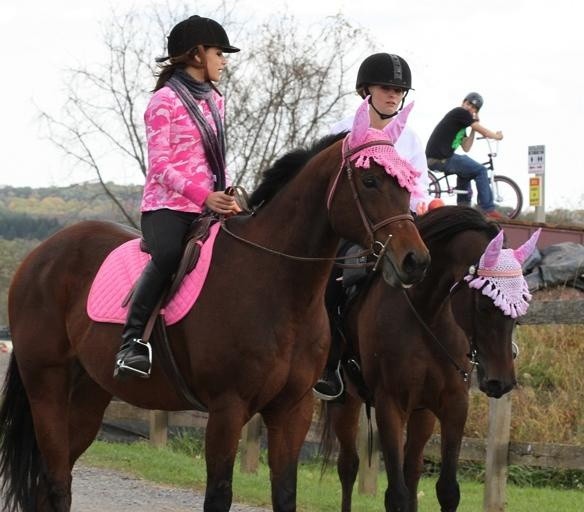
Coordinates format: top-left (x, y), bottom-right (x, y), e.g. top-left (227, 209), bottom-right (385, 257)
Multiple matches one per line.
top-left (314, 337), bottom-right (339, 395)
top-left (112, 257), bottom-right (168, 382)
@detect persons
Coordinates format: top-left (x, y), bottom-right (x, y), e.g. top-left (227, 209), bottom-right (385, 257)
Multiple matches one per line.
top-left (310, 52), bottom-right (417, 401)
top-left (425, 93), bottom-right (504, 221)
top-left (113, 15), bottom-right (237, 377)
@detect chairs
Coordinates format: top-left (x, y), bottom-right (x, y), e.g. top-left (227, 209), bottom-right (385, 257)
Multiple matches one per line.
top-left (483, 208), bottom-right (513, 223)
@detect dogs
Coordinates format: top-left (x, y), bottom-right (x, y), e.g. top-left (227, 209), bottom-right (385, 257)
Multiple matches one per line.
top-left (315, 205), bottom-right (543, 512)
top-left (0, 94), bottom-right (432, 512)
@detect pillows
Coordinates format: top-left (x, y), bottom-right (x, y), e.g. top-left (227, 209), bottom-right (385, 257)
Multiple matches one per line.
top-left (355, 53), bottom-right (411, 101)
top-left (168, 15), bottom-right (240, 64)
top-left (464, 93), bottom-right (483, 114)
